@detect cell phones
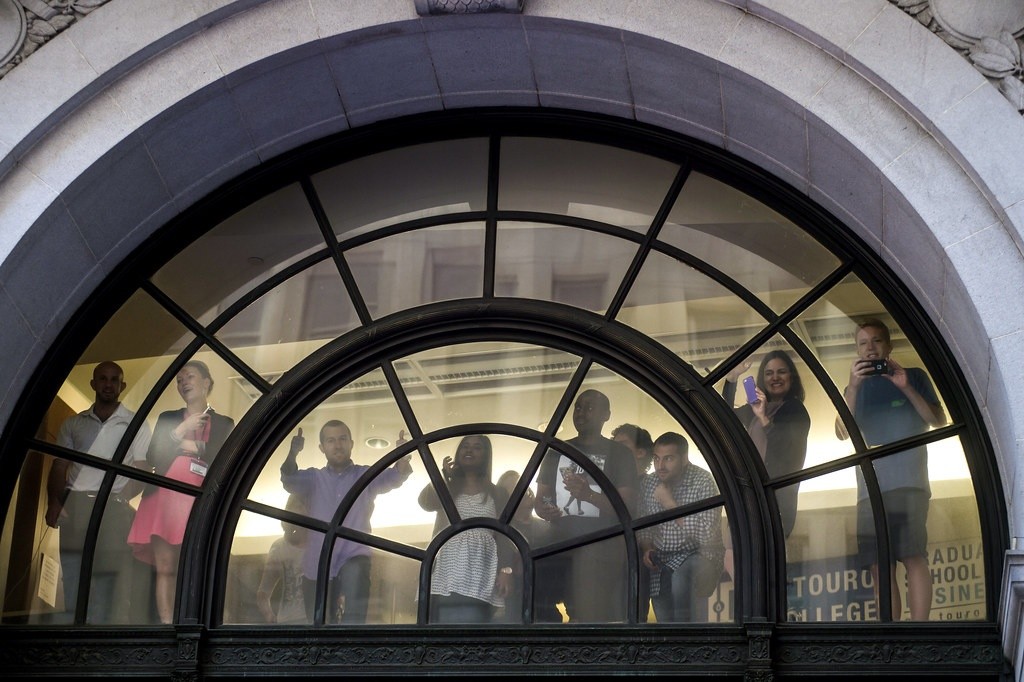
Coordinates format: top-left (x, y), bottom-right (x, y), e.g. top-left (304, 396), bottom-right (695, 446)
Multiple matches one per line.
top-left (201, 407), bottom-right (214, 421)
top-left (857, 359), bottom-right (887, 375)
top-left (743, 376), bottom-right (759, 404)
top-left (560, 467), bottom-right (574, 481)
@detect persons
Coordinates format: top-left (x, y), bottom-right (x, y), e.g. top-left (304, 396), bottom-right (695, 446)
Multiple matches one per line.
top-left (722, 349), bottom-right (810, 591)
top-left (835, 319), bottom-right (948, 621)
top-left (256, 420), bottom-right (413, 625)
top-left (126, 360), bottom-right (235, 625)
top-left (45, 360), bottom-right (149, 625)
top-left (417, 388), bottom-right (733, 624)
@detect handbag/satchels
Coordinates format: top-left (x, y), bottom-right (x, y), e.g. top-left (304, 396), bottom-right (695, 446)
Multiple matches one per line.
top-left (92, 496), bottom-right (138, 572)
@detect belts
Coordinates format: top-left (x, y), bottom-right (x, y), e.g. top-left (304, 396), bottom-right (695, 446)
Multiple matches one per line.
top-left (68, 491), bottom-right (117, 497)
